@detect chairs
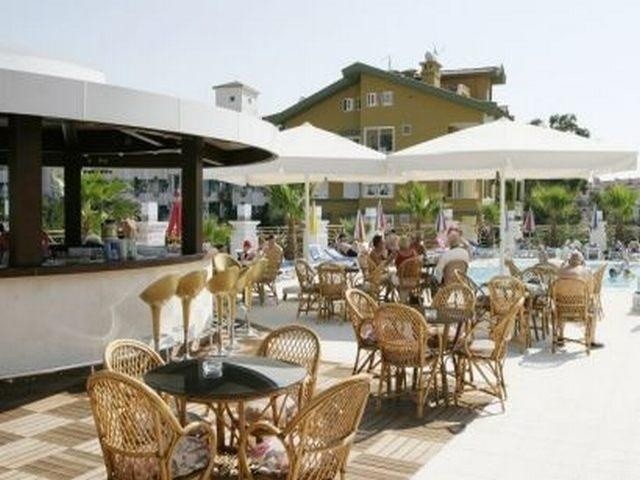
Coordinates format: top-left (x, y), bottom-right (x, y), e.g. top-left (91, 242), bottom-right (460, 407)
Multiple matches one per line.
top-left (100, 338), bottom-right (172, 407)
top-left (507, 254), bottom-right (607, 354)
top-left (237, 372), bottom-right (373, 478)
top-left (295, 259), bottom-right (348, 322)
top-left (345, 256), bottom-right (523, 421)
top-left (87, 368), bottom-right (219, 479)
top-left (234, 323), bottom-right (322, 431)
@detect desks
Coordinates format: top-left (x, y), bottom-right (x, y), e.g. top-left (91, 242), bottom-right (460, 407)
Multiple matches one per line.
top-left (144, 354), bottom-right (306, 480)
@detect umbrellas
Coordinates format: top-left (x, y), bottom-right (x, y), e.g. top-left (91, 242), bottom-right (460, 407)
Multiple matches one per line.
top-left (204, 121), bottom-right (390, 227)
top-left (376, 110), bottom-right (637, 264)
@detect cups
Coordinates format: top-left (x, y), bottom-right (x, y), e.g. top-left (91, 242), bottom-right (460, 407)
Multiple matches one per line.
top-left (202, 361), bottom-right (223, 380)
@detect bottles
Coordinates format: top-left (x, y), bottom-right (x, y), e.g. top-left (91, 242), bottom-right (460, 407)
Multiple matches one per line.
top-left (44, 218), bottom-right (137, 264)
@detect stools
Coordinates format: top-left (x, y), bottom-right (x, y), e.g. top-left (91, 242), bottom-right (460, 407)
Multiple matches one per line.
top-left (139, 242), bottom-right (282, 361)
